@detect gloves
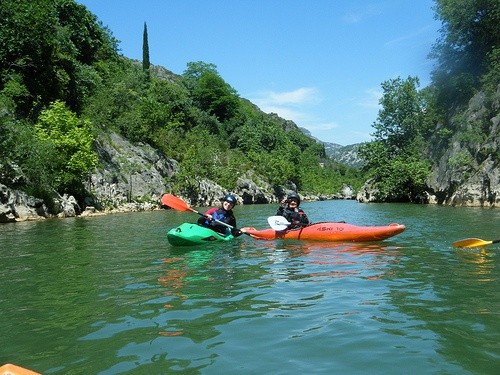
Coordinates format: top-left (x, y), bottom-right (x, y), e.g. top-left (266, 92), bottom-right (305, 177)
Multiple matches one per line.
top-left (231, 228), bottom-right (242, 237)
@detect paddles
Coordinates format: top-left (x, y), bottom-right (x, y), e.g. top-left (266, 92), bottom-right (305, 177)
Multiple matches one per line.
top-left (160, 193), bottom-right (276, 241)
top-left (267, 215), bottom-right (311, 231)
top-left (453, 237), bottom-right (500, 249)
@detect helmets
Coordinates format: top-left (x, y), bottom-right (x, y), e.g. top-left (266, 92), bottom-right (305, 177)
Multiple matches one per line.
top-left (287, 194), bottom-right (300, 203)
top-left (224, 195), bottom-right (237, 205)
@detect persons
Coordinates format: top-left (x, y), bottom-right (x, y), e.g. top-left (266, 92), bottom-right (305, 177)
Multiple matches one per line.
top-left (274, 191), bottom-right (310, 231)
top-left (196, 194), bottom-right (237, 235)
